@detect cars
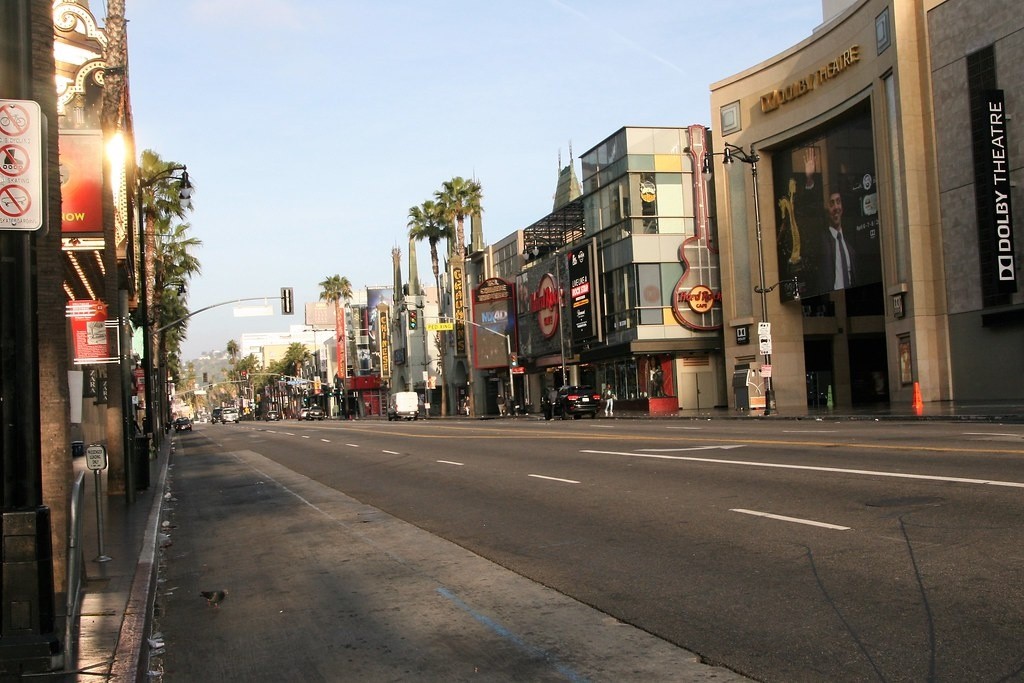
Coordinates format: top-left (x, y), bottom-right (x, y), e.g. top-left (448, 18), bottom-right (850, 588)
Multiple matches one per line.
top-left (298, 407), bottom-right (309, 421)
top-left (266, 410), bottom-right (279, 421)
top-left (211, 406), bottom-right (240, 425)
top-left (306, 406), bottom-right (325, 421)
top-left (172, 417), bottom-right (192, 433)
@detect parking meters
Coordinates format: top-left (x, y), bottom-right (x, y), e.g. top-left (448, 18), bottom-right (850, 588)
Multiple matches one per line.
top-left (85, 444), bottom-right (113, 563)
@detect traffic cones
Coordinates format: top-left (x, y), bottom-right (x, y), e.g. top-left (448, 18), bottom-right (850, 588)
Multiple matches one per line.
top-left (912, 381), bottom-right (925, 409)
top-left (912, 407), bottom-right (922, 416)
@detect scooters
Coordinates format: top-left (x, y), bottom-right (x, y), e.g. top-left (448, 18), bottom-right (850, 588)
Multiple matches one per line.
top-left (211, 413), bottom-right (219, 425)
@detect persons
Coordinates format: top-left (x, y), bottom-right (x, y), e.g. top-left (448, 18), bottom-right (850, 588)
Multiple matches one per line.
top-left (648, 356), bottom-right (663, 398)
top-left (602, 383), bottom-right (616, 416)
top-left (463, 395), bottom-right (470, 417)
top-left (132, 414), bottom-right (141, 436)
top-left (798, 148), bottom-right (868, 294)
top-left (497, 385), bottom-right (562, 421)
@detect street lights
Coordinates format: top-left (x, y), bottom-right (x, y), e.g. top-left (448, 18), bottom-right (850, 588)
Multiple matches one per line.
top-left (699, 142), bottom-right (801, 418)
top-left (521, 235), bottom-right (567, 388)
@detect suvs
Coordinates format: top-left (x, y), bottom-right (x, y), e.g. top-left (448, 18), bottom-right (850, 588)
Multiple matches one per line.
top-left (545, 385), bottom-right (601, 419)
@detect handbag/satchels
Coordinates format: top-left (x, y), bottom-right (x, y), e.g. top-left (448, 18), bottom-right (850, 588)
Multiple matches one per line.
top-left (612, 394), bottom-right (617, 401)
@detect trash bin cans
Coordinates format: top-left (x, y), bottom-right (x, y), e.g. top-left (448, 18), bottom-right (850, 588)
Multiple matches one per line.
top-left (134, 437), bottom-right (152, 491)
top-left (72, 441), bottom-right (84, 457)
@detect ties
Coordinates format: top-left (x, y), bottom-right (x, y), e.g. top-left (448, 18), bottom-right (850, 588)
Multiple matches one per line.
top-left (836, 232), bottom-right (850, 288)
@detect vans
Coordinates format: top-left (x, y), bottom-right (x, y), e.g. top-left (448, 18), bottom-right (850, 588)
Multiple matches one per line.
top-left (387, 392), bottom-right (420, 422)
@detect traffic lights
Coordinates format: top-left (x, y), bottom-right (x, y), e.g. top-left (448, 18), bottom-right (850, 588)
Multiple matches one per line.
top-left (242, 370), bottom-right (246, 376)
top-left (280, 372), bottom-right (284, 381)
top-left (338, 385), bottom-right (343, 395)
top-left (408, 310), bottom-right (418, 330)
top-left (328, 392), bottom-right (334, 397)
